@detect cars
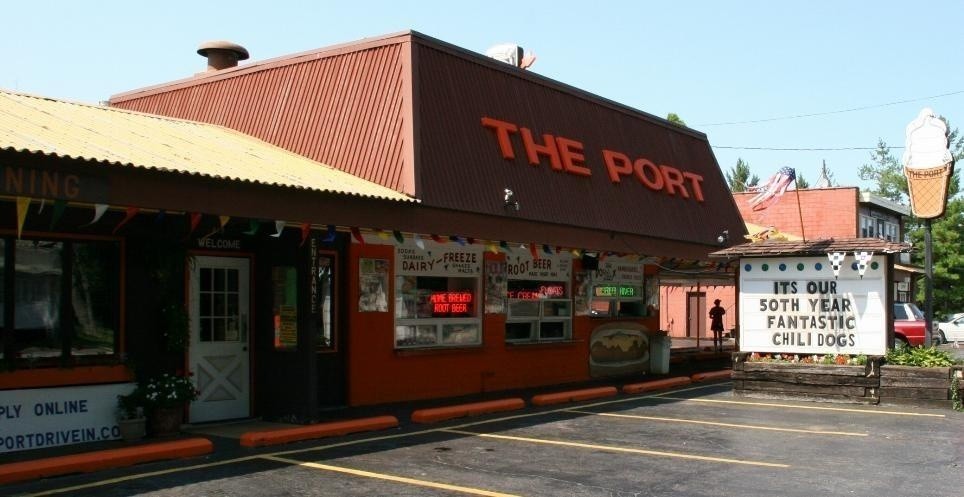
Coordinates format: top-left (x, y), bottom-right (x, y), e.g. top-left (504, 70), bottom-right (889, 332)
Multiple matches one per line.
top-left (935, 310), bottom-right (964, 344)
top-left (892, 297), bottom-right (941, 352)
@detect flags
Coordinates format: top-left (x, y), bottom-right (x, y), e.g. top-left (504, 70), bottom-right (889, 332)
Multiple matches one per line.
top-left (734, 166), bottom-right (796, 212)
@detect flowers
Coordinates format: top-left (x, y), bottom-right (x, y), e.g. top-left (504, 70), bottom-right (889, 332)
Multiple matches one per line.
top-left (145, 371), bottom-right (200, 408)
top-left (744, 351), bottom-right (868, 366)
top-left (655, 330), bottom-right (667, 336)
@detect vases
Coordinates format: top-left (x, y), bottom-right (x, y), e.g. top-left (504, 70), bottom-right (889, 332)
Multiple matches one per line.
top-left (150, 404), bottom-right (178, 435)
top-left (729, 350), bottom-right (884, 404)
top-left (649, 335), bottom-right (671, 375)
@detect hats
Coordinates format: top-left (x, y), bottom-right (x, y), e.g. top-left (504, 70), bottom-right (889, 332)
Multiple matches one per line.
top-left (714, 299), bottom-right (721, 304)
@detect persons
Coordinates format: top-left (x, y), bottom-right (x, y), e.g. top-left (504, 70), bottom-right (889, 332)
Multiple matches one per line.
top-left (708, 298), bottom-right (726, 352)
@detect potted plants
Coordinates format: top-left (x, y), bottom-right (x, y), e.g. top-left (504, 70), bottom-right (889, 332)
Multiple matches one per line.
top-left (116, 385), bottom-right (152, 442)
top-left (879, 342), bottom-right (962, 411)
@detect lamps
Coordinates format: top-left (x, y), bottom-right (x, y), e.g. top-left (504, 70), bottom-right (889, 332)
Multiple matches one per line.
top-left (717, 229), bottom-right (729, 249)
top-left (503, 188), bottom-right (519, 211)
